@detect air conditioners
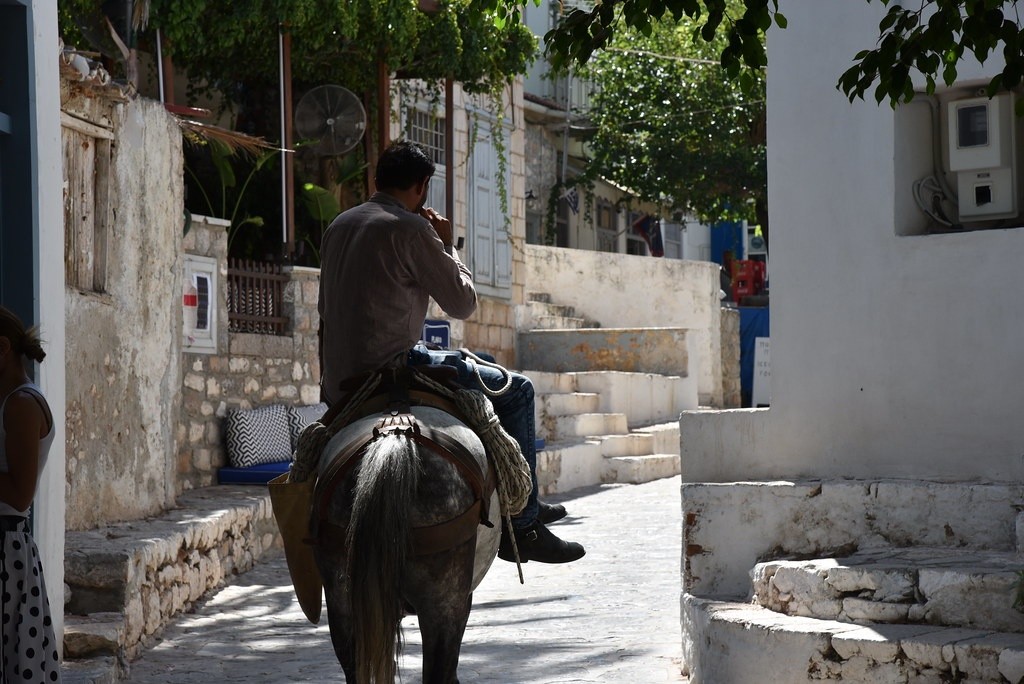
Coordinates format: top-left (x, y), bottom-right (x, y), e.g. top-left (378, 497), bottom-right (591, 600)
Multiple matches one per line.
top-left (747, 234), bottom-right (767, 252)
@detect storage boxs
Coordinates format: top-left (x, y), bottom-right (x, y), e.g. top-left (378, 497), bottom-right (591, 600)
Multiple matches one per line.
top-left (733, 278), bottom-right (762, 302)
top-left (731, 260), bottom-right (762, 279)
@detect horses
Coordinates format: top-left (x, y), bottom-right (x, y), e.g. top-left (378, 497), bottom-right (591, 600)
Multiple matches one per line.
top-left (309, 404), bottom-right (501, 684)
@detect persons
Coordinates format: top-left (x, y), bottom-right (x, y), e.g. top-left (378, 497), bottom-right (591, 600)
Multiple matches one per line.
top-left (317, 142), bottom-right (586, 563)
top-left (0, 306), bottom-right (60, 684)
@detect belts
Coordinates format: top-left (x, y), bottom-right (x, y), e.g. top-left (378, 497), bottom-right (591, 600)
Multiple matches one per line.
top-left (0, 515), bottom-right (31, 532)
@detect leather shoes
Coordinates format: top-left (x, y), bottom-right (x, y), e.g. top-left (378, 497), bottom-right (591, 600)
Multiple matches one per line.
top-left (543, 504), bottom-right (568, 524)
top-left (498, 519), bottom-right (586, 563)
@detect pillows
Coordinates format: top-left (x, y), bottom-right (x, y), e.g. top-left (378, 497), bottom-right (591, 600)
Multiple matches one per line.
top-left (286, 402), bottom-right (329, 453)
top-left (226, 403), bottom-right (292, 468)
top-left (227, 283), bottom-right (278, 335)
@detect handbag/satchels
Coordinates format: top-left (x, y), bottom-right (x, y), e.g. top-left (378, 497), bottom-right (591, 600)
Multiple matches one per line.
top-left (268, 471), bottom-right (322, 624)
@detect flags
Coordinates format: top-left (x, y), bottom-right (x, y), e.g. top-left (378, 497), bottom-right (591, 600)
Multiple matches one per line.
top-left (638, 216), bottom-right (663, 257)
